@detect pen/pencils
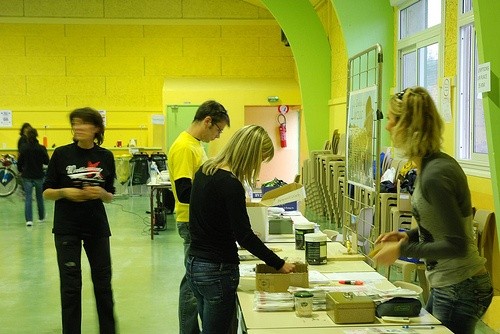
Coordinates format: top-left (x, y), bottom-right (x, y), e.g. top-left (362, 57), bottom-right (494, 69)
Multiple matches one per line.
top-left (339, 280), bottom-right (363, 285)
top-left (402, 326), bottom-right (434, 329)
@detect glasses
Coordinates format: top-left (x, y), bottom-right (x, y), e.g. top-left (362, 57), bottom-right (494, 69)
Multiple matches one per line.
top-left (212, 120), bottom-right (224, 135)
top-left (394, 88), bottom-right (414, 99)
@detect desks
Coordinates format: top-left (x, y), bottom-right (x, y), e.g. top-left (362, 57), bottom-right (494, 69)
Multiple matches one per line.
top-left (234, 209), bottom-right (455, 334)
top-left (146, 169), bottom-right (172, 240)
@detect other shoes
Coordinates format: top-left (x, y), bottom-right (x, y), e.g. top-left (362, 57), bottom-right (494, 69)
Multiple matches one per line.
top-left (38, 217), bottom-right (48, 222)
top-left (26, 221), bottom-right (33, 226)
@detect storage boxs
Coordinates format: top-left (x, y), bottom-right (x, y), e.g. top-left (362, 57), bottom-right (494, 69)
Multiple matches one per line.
top-left (325, 292), bottom-right (376, 324)
top-left (255, 261), bottom-right (309, 293)
top-left (235, 174), bottom-right (307, 245)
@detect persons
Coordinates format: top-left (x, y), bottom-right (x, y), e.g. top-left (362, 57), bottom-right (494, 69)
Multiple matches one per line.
top-left (168, 101), bottom-right (231, 334)
top-left (17, 129), bottom-right (49, 226)
top-left (18, 122), bottom-right (38, 154)
top-left (370, 87), bottom-right (494, 334)
top-left (41, 107), bottom-right (116, 334)
top-left (184, 125), bottom-right (297, 334)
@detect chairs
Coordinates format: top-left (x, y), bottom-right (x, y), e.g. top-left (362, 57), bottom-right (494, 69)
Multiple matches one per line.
top-left (472, 209), bottom-right (494, 258)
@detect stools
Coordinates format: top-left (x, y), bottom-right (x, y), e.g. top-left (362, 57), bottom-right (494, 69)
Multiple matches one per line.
top-left (301, 149), bottom-right (426, 306)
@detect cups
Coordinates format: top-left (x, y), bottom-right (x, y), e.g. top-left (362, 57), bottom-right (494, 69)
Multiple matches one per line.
top-left (294, 291), bottom-right (314, 316)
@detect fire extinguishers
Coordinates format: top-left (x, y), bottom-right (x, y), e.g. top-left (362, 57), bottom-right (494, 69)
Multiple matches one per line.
top-left (278, 114), bottom-right (287, 147)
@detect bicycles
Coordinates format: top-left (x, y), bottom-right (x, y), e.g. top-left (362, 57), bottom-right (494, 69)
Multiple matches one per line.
top-left (0, 153), bottom-right (48, 197)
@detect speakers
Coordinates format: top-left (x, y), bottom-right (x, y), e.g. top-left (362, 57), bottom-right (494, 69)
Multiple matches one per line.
top-left (151, 154), bottom-right (167, 172)
top-left (129, 154), bottom-right (149, 185)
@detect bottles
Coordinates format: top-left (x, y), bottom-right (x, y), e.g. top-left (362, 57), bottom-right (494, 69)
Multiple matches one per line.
top-left (150, 161), bottom-right (157, 184)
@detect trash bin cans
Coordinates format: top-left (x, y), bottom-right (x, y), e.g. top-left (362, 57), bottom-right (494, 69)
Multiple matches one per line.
top-left (149, 152), bottom-right (167, 172)
top-left (128, 152), bottom-right (149, 186)
